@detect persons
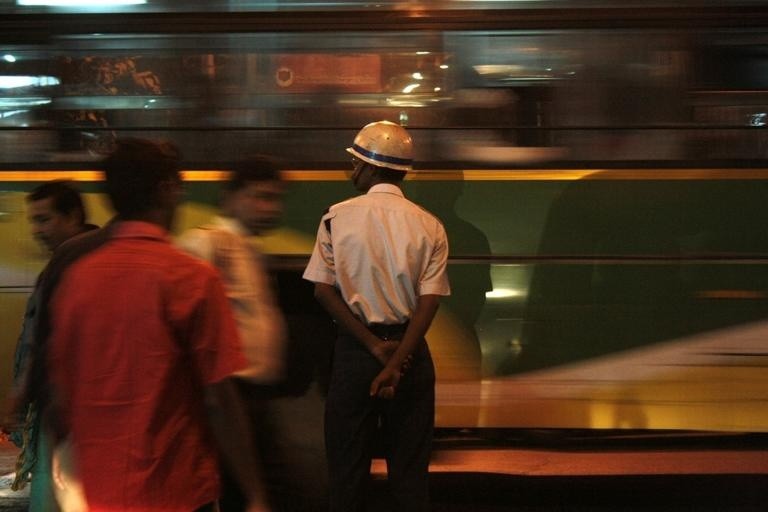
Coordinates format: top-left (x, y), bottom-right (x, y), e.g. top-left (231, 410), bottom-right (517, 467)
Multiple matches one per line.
top-left (41, 138), bottom-right (272, 512)
top-left (0, 180), bottom-right (102, 512)
top-left (172, 147), bottom-right (292, 512)
top-left (301, 118), bottom-right (452, 512)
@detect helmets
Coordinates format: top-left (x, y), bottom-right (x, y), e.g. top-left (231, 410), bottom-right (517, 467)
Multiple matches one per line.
top-left (347, 120), bottom-right (414, 172)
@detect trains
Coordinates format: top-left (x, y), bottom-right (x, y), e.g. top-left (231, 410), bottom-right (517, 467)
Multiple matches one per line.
top-left (0, 0), bottom-right (768, 512)
